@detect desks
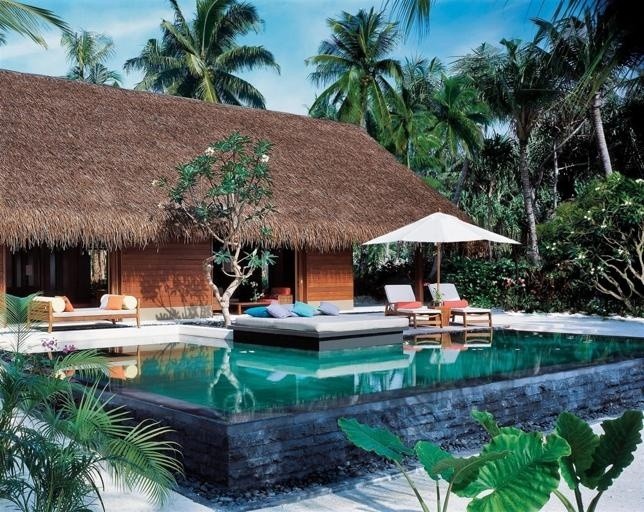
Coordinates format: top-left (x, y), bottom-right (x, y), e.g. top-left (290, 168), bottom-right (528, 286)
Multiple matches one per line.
top-left (228, 301), bottom-right (270, 315)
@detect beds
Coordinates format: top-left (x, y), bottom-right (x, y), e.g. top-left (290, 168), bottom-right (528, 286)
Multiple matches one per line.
top-left (229, 313), bottom-right (410, 353)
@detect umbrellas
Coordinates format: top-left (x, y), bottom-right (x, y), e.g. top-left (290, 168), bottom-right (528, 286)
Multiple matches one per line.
top-left (360, 208), bottom-right (522, 302)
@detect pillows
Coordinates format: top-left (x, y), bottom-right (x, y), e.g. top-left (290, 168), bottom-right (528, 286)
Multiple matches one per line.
top-left (105, 364), bottom-right (139, 381)
top-left (99, 294), bottom-right (137, 311)
top-left (31, 294), bottom-right (74, 312)
top-left (243, 299), bottom-right (340, 320)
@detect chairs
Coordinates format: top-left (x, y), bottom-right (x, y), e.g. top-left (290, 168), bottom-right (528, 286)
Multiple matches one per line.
top-left (385, 283), bottom-right (493, 327)
top-left (412, 328), bottom-right (493, 350)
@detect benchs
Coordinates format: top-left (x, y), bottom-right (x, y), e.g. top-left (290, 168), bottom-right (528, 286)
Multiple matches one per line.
top-left (47, 346), bottom-right (144, 381)
top-left (26, 298), bottom-right (142, 333)
